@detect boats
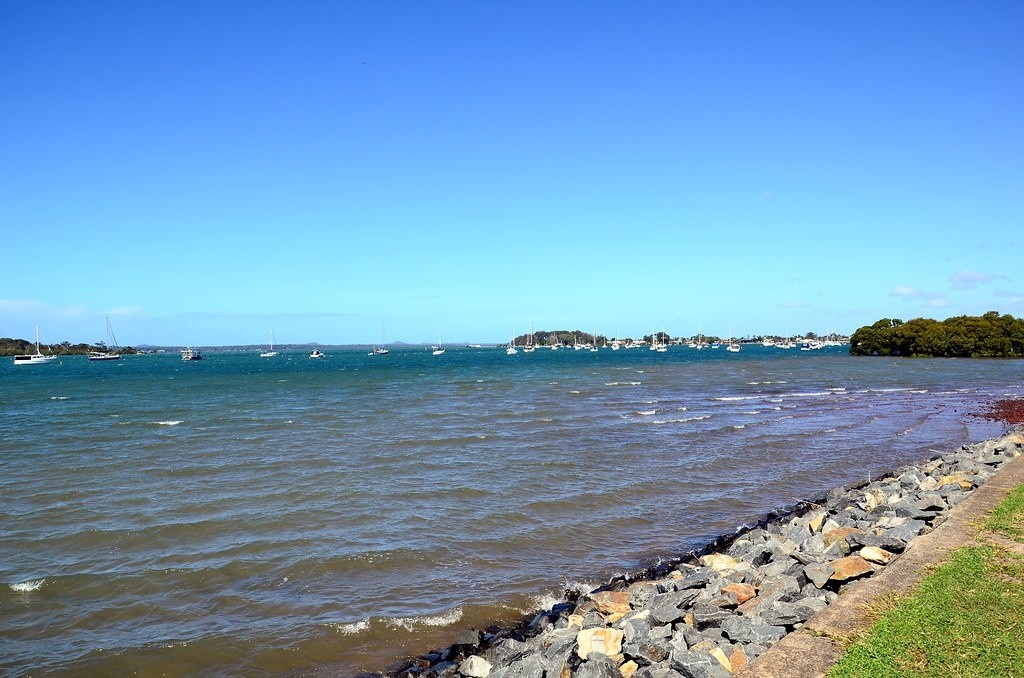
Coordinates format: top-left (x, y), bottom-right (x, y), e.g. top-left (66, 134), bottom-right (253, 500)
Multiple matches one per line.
top-left (309, 349), bottom-right (326, 359)
top-left (87, 317), bottom-right (121, 360)
top-left (801, 332), bottom-right (846, 351)
top-left (14, 324), bottom-right (60, 366)
top-left (762, 332), bottom-right (797, 349)
top-left (625, 338), bottom-right (641, 348)
top-left (727, 325), bottom-right (740, 352)
top-left (551, 329), bottom-right (598, 352)
top-left (431, 335), bottom-right (445, 356)
top-left (712, 341), bottom-right (719, 348)
top-left (611, 333), bottom-right (619, 351)
top-left (687, 321), bottom-right (710, 351)
top-left (181, 348), bottom-right (202, 360)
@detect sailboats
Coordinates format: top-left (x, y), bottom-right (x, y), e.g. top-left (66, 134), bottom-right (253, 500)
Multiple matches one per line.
top-left (367, 316), bottom-right (388, 356)
top-left (506, 328), bottom-right (518, 355)
top-left (523, 321), bottom-right (540, 352)
top-left (650, 327), bottom-right (668, 352)
top-left (260, 325), bottom-right (279, 358)
top-left (602, 336), bottom-right (607, 348)
